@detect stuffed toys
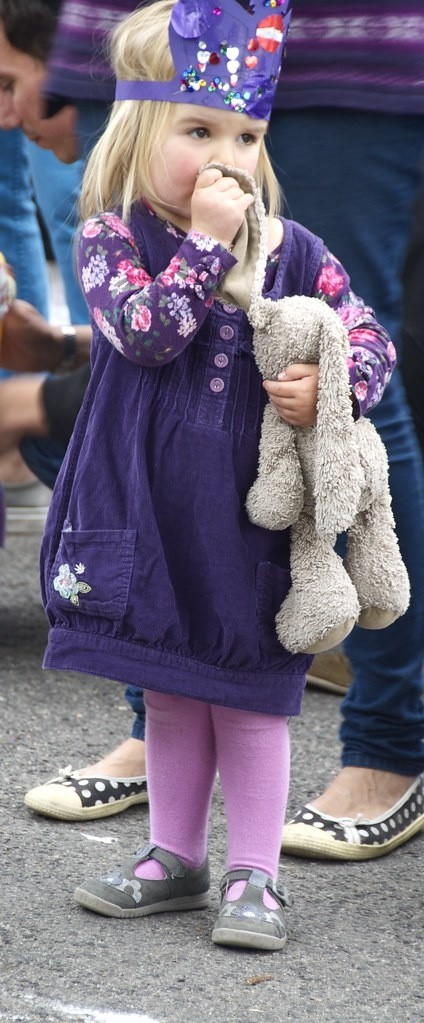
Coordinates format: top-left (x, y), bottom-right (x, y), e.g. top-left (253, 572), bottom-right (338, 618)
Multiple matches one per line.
top-left (199, 161), bottom-right (410, 658)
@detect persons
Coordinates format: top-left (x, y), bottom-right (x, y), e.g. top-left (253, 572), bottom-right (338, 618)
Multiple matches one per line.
top-left (0, 129), bottom-right (94, 380)
top-left (40, 1), bottom-right (396, 955)
top-left (0, 0), bottom-right (93, 488)
top-left (23, 0), bottom-right (424, 864)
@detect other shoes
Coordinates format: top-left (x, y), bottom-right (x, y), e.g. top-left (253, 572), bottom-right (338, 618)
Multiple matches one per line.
top-left (212, 870), bottom-right (291, 950)
top-left (305, 651), bottom-right (354, 693)
top-left (280, 772), bottom-right (424, 862)
top-left (74, 845), bottom-right (209, 918)
top-left (22, 765), bottom-right (147, 821)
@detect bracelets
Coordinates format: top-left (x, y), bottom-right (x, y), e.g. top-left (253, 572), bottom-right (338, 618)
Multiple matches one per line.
top-left (61, 325), bottom-right (77, 357)
